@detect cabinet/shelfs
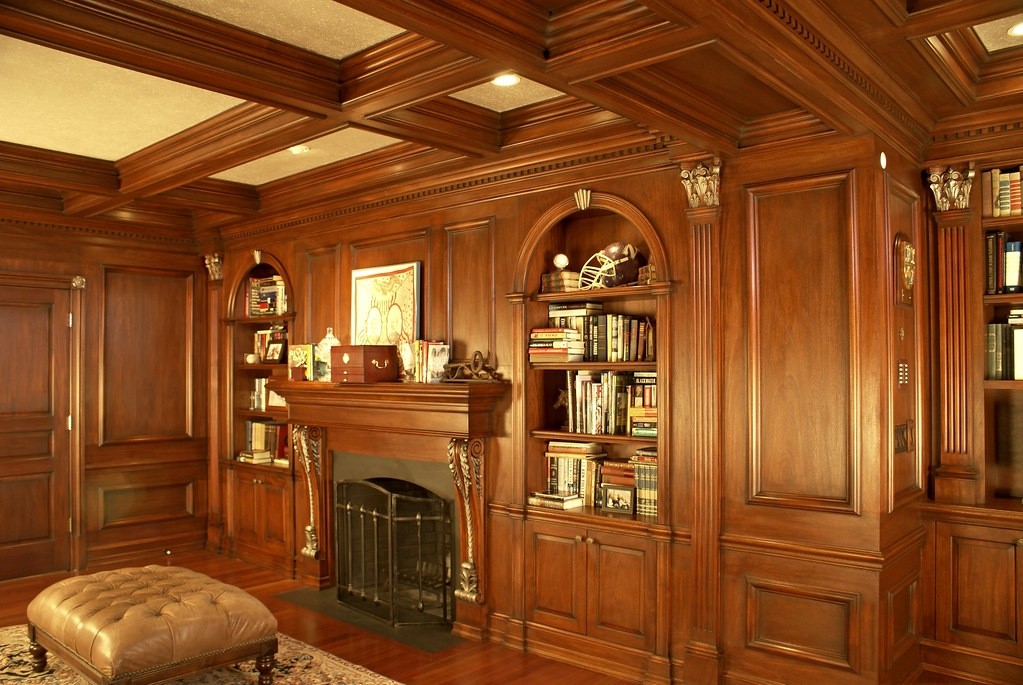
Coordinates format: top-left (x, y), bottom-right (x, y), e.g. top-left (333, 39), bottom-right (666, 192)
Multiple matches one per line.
top-left (935, 146), bottom-right (1023, 666)
top-left (503, 189), bottom-right (684, 685)
top-left (221, 248), bottom-right (297, 581)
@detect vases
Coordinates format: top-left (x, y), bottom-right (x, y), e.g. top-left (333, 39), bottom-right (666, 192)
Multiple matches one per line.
top-left (291, 366), bottom-right (307, 381)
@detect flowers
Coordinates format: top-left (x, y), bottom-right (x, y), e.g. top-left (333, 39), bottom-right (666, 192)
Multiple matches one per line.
top-left (288, 348), bottom-right (308, 367)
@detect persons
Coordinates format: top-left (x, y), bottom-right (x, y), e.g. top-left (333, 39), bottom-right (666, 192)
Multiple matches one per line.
top-left (607, 493), bottom-right (629, 509)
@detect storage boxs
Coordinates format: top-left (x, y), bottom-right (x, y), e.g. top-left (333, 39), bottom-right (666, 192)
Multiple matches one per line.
top-left (330, 345), bottom-right (399, 383)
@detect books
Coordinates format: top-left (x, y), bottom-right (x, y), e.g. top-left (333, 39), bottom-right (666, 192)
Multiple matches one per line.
top-left (529, 270), bottom-right (657, 516)
top-left (415, 337), bottom-right (450, 384)
top-left (238, 273), bottom-right (288, 470)
top-left (287, 343), bottom-right (313, 382)
top-left (981, 164), bottom-right (1023, 381)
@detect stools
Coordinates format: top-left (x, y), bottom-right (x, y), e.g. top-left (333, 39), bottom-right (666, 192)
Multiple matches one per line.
top-left (27, 563), bottom-right (279, 685)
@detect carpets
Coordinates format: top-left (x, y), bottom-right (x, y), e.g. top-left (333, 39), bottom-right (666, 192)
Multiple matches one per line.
top-left (0, 622), bottom-right (408, 685)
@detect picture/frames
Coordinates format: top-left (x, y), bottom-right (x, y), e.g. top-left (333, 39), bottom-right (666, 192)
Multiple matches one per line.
top-left (262, 339), bottom-right (287, 364)
top-left (601, 485), bottom-right (635, 515)
top-left (265, 376), bottom-right (289, 412)
top-left (350, 259), bottom-right (421, 382)
